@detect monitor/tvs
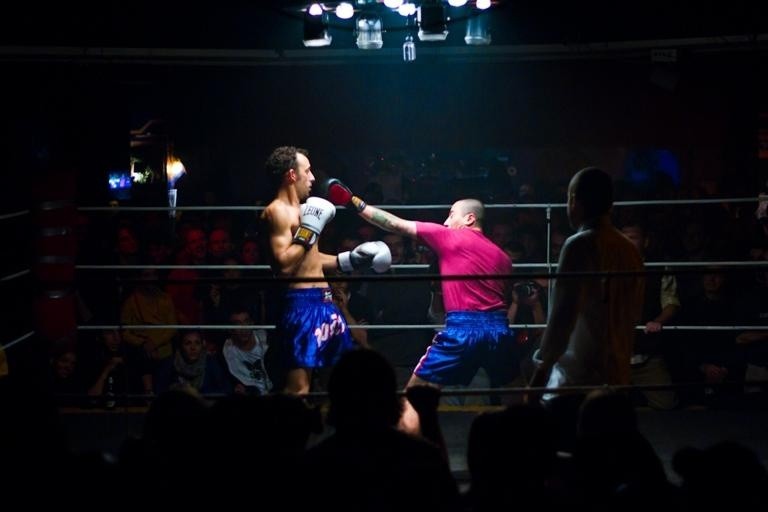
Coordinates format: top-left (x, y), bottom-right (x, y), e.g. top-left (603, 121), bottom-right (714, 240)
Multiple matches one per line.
top-left (106, 169), bottom-right (135, 192)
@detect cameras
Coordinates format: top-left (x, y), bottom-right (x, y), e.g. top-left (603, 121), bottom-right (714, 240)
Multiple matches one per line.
top-left (515, 280), bottom-right (542, 296)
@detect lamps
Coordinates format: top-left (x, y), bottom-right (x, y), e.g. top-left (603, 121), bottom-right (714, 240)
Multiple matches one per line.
top-left (302, 5), bottom-right (332, 47)
top-left (403, 42), bottom-right (416, 61)
top-left (356, 19), bottom-right (384, 49)
top-left (417, 0), bottom-right (448, 41)
top-left (465, 13), bottom-right (492, 46)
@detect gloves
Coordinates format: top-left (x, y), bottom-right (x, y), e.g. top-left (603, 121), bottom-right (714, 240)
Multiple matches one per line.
top-left (330, 238), bottom-right (394, 278)
top-left (316, 171), bottom-right (367, 214)
top-left (289, 195), bottom-right (338, 251)
